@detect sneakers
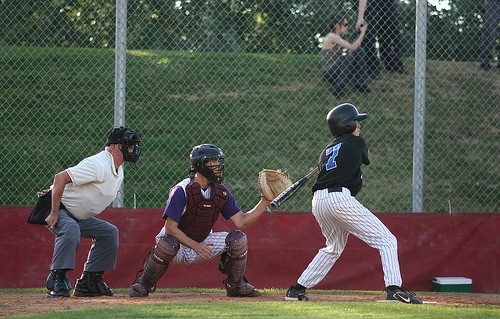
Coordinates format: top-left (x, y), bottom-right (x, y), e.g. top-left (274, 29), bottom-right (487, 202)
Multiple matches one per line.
top-left (73, 279), bottom-right (113, 296)
top-left (228, 287), bottom-right (261, 297)
top-left (47, 275), bottom-right (71, 297)
top-left (284, 285), bottom-right (308, 301)
top-left (387, 290), bottom-right (422, 305)
top-left (129, 288), bottom-right (148, 298)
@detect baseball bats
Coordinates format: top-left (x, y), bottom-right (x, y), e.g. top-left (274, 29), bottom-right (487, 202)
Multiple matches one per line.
top-left (269, 165), bottom-right (320, 210)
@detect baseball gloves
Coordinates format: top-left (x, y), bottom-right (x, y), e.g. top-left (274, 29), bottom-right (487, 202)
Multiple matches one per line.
top-left (257, 169), bottom-right (294, 201)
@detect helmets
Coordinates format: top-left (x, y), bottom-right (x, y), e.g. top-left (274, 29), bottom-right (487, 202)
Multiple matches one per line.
top-left (190, 143), bottom-right (224, 184)
top-left (327, 104), bottom-right (366, 135)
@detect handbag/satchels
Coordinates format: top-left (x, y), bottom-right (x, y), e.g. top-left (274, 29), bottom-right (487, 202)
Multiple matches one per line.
top-left (28, 190), bottom-right (52, 224)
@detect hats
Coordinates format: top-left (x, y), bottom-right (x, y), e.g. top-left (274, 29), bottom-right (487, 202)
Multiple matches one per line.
top-left (107, 129), bottom-right (126, 144)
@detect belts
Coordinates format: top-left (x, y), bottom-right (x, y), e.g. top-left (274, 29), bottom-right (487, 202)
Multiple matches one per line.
top-left (328, 187), bottom-right (341, 192)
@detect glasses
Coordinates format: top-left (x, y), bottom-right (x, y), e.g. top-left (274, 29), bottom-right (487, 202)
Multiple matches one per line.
top-left (341, 23), bottom-right (350, 26)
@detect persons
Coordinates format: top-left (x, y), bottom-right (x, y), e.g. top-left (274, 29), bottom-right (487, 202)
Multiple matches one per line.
top-left (129, 143), bottom-right (293, 298)
top-left (27, 126), bottom-right (142, 297)
top-left (284, 103), bottom-right (424, 305)
top-left (480, 0), bottom-right (500, 70)
top-left (322, 14), bottom-right (371, 97)
top-left (351, 0), bottom-right (409, 79)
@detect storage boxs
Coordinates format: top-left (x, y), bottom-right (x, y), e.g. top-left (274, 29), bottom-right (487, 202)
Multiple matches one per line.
top-left (432, 277), bottom-right (472, 293)
